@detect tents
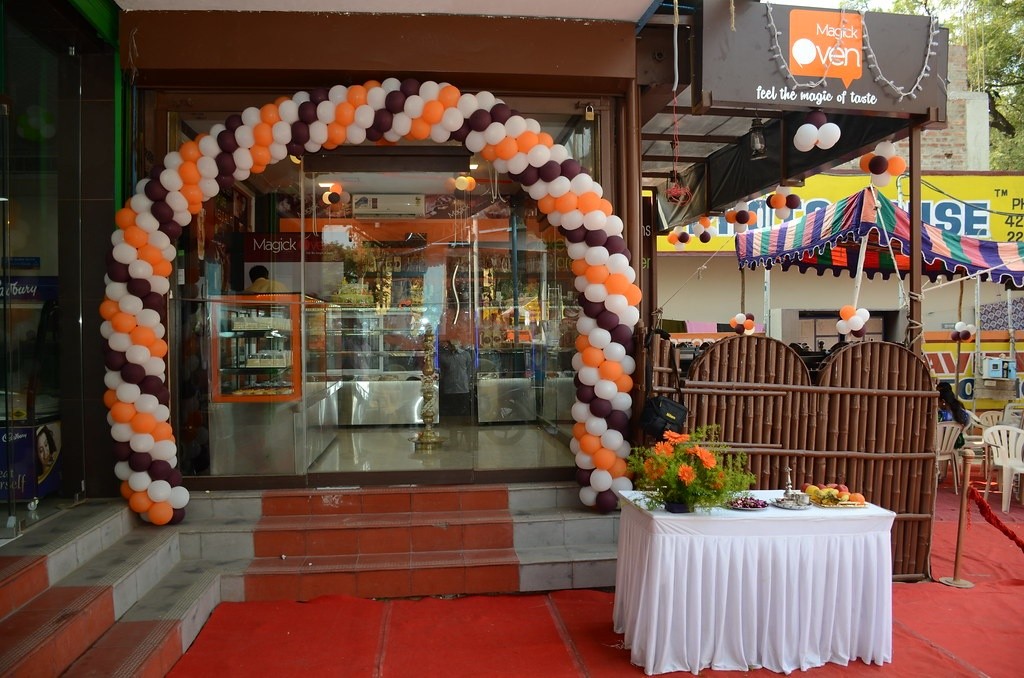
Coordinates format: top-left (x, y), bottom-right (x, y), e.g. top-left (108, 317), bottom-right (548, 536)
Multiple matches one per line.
top-left (736, 187), bottom-right (1024, 375)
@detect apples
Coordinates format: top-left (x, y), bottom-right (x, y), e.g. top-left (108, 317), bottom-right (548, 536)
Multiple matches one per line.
top-left (802, 483), bottom-right (848, 498)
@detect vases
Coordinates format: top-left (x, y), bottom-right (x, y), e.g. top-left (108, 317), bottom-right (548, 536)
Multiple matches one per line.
top-left (664, 500), bottom-right (694, 513)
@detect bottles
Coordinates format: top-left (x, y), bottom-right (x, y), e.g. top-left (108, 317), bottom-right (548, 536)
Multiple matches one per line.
top-left (239, 309), bottom-right (265, 318)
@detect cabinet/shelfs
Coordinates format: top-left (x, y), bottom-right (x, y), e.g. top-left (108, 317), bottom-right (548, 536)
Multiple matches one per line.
top-left (769, 309), bottom-right (909, 353)
top-left (207, 246), bottom-right (574, 477)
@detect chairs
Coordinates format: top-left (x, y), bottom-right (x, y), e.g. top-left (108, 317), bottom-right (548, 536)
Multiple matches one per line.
top-left (937, 404), bottom-right (1024, 514)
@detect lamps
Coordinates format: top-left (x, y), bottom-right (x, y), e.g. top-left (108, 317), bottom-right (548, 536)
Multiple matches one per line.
top-left (750, 114), bottom-right (770, 161)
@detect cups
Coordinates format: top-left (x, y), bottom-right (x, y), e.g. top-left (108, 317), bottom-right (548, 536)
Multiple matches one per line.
top-left (795, 493), bottom-right (809, 506)
top-left (775, 497), bottom-right (794, 506)
top-left (250, 337), bottom-right (256, 354)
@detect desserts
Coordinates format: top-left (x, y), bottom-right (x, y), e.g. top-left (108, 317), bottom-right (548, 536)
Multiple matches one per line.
top-left (233, 315), bottom-right (293, 367)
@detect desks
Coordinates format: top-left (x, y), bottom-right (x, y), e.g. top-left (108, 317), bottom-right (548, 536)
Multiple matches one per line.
top-left (615, 489), bottom-right (897, 676)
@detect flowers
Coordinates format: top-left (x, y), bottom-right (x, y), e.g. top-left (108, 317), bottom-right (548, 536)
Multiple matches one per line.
top-left (623, 425), bottom-right (756, 514)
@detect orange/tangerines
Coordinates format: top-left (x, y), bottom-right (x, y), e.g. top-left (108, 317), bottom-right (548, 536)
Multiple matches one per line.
top-left (837, 491), bottom-right (865, 505)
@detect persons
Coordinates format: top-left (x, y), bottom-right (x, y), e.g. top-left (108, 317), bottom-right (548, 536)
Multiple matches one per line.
top-left (35, 425), bottom-right (57, 476)
top-left (241, 265), bottom-right (293, 294)
top-left (438, 335), bottom-right (472, 451)
top-left (343, 323), bottom-right (371, 369)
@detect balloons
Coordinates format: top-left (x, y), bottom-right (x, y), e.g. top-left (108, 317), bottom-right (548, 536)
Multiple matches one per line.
top-left (766, 184), bottom-right (800, 219)
top-left (667, 226), bottom-right (692, 250)
top-left (446, 175), bottom-right (476, 199)
top-left (951, 322), bottom-right (976, 343)
top-left (860, 142), bottom-right (906, 187)
top-left (730, 313), bottom-right (755, 335)
top-left (100, 78), bottom-right (643, 526)
top-left (794, 111), bottom-right (840, 151)
top-left (693, 217), bottom-right (717, 243)
top-left (725, 201), bottom-right (757, 234)
top-left (323, 183), bottom-right (350, 211)
top-left (836, 305), bottom-right (870, 338)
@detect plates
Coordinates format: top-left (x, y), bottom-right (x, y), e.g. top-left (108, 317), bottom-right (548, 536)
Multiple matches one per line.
top-left (769, 497), bottom-right (813, 510)
top-left (725, 503), bottom-right (768, 510)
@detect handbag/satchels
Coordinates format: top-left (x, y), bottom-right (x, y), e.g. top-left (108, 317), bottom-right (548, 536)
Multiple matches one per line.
top-left (638, 396), bottom-right (690, 440)
